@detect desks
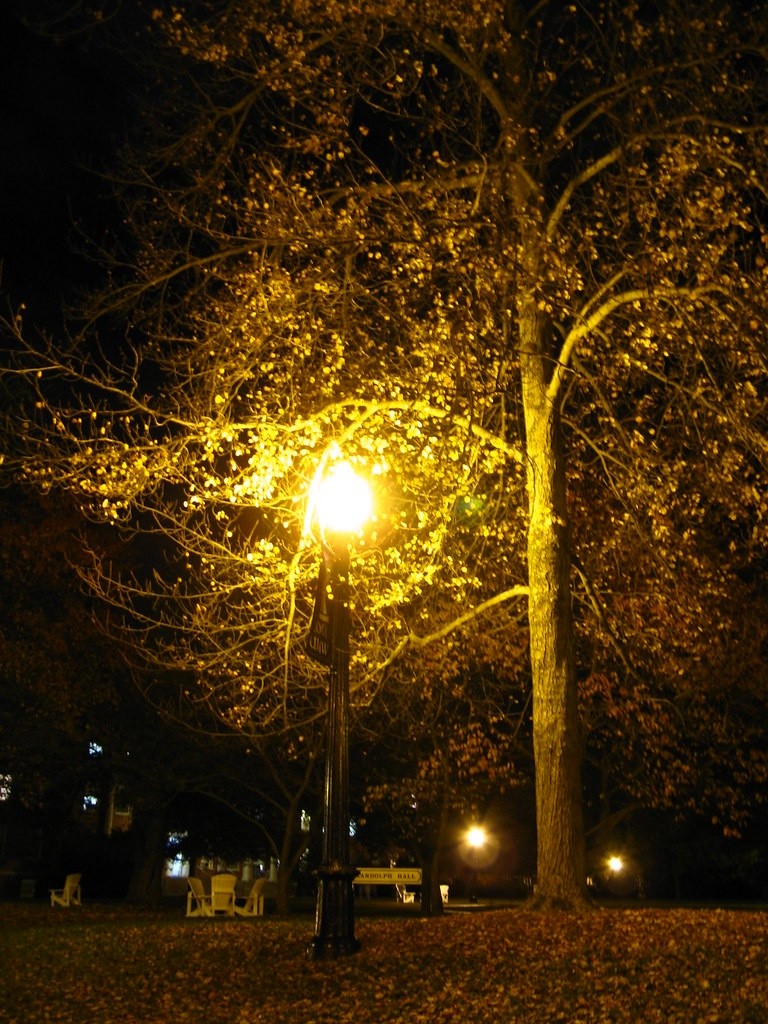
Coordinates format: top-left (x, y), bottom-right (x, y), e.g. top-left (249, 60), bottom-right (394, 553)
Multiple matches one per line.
top-left (191, 895), bottom-right (254, 917)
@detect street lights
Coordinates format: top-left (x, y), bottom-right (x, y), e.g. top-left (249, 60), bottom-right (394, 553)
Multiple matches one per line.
top-left (305, 460), bottom-right (373, 959)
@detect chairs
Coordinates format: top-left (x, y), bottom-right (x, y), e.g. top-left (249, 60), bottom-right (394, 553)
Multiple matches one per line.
top-left (185, 873), bottom-right (266, 917)
top-left (48, 873), bottom-right (82, 907)
